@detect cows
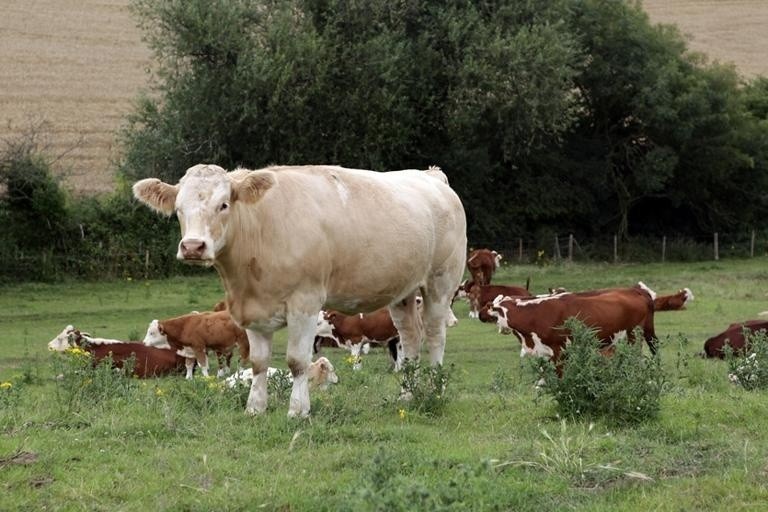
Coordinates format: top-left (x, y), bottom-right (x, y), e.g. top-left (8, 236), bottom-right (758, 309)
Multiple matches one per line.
top-left (394, 296), bottom-right (458, 373)
top-left (131, 162), bottom-right (469, 419)
top-left (141, 309), bottom-right (250, 381)
top-left (315, 304), bottom-right (399, 373)
top-left (703, 319), bottom-right (768, 359)
top-left (465, 281), bottom-right (660, 390)
top-left (466, 248), bottom-right (503, 284)
top-left (202, 296), bottom-right (234, 379)
top-left (215, 356), bottom-right (340, 393)
top-left (653, 287), bottom-right (694, 311)
top-left (45, 324), bottom-right (198, 378)
